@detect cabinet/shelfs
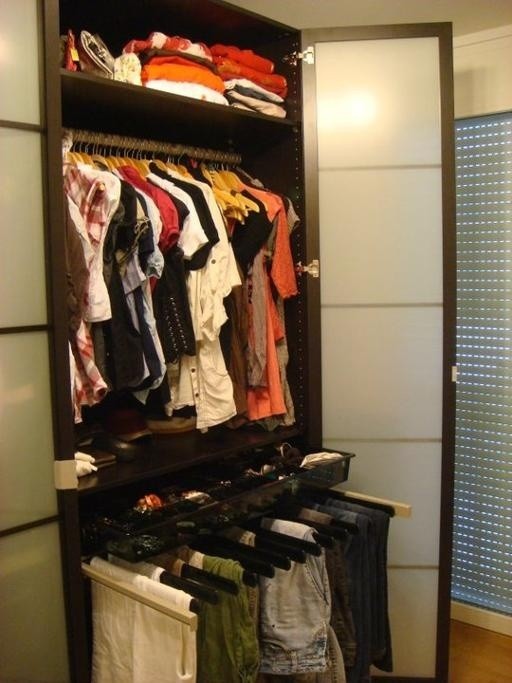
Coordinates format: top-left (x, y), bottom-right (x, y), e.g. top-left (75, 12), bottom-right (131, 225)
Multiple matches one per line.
top-left (2, 0), bottom-right (454, 675)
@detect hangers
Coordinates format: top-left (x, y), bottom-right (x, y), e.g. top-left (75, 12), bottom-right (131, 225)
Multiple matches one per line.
top-left (63, 128), bottom-right (269, 214)
